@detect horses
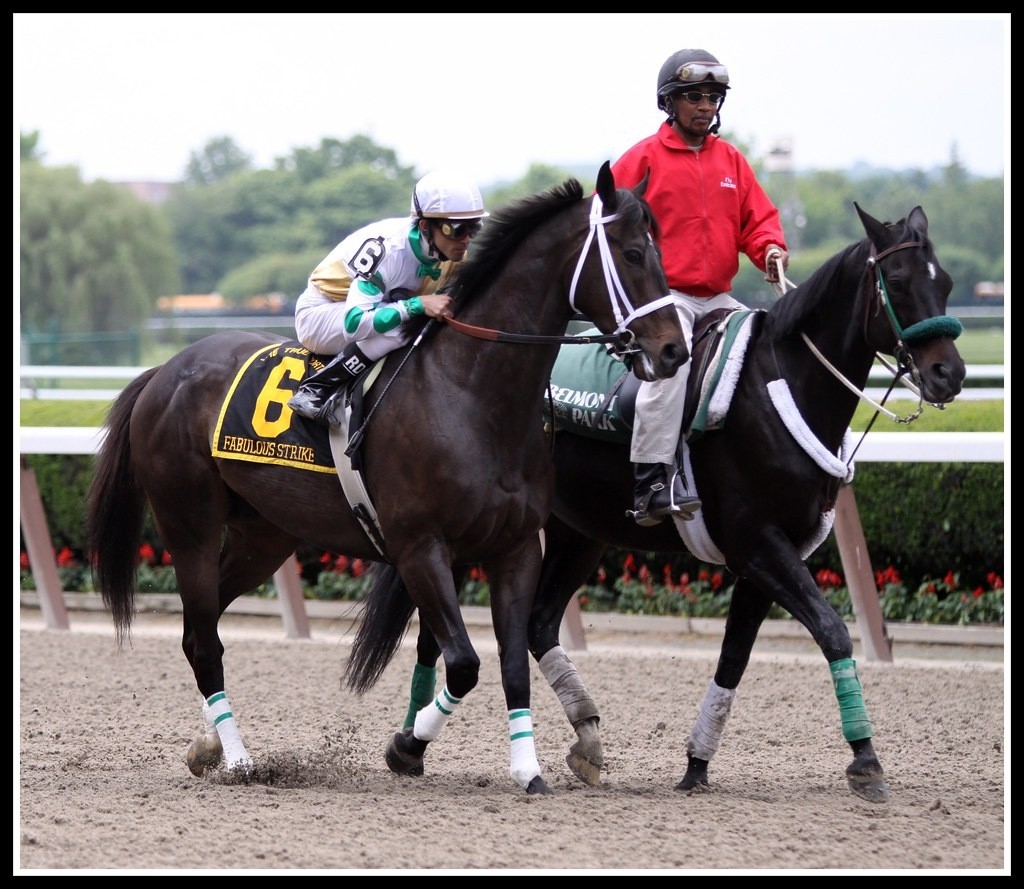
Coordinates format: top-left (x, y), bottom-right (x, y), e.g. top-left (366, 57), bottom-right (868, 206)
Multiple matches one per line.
top-left (89, 160), bottom-right (968, 808)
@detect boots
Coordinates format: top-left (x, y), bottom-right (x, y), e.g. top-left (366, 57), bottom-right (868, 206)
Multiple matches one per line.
top-left (287, 341), bottom-right (375, 418)
top-left (635, 463), bottom-right (702, 526)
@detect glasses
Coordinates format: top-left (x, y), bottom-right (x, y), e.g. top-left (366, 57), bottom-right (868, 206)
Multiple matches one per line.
top-left (429, 220), bottom-right (482, 241)
top-left (676, 61), bottom-right (730, 84)
top-left (672, 92), bottom-right (723, 105)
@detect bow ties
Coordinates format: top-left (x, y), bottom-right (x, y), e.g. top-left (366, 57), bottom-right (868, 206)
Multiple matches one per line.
top-left (408, 223), bottom-right (443, 282)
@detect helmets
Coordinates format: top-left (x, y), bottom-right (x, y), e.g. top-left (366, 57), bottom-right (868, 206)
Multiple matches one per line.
top-left (657, 49), bottom-right (731, 111)
top-left (410, 169), bottom-right (490, 219)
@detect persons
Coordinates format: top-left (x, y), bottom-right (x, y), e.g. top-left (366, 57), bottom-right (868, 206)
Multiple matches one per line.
top-left (287, 169), bottom-right (490, 419)
top-left (593, 48), bottom-right (790, 527)
